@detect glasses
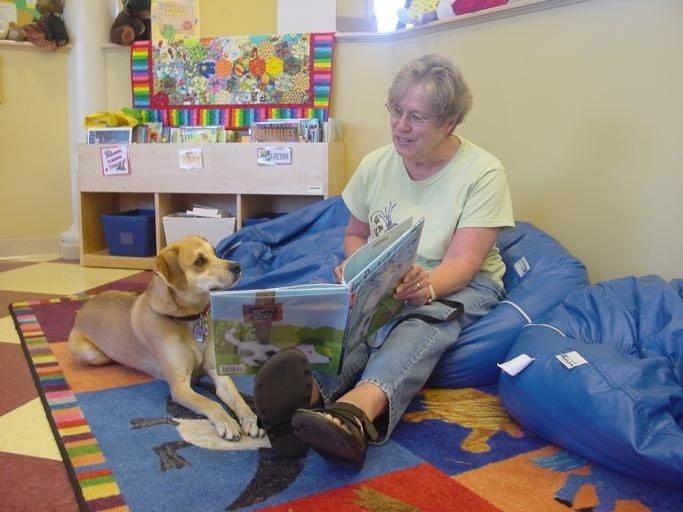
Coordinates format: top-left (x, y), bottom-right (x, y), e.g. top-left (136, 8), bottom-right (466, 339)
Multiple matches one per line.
top-left (384, 104), bottom-right (437, 126)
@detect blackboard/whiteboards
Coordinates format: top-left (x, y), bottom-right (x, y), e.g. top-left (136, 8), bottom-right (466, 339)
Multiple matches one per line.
top-left (88, 127), bottom-right (133, 144)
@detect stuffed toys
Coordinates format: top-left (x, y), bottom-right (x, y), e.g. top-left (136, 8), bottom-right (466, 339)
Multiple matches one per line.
top-left (0, 19), bottom-right (25, 42)
top-left (109, 0), bottom-right (151, 45)
top-left (20, 0), bottom-right (67, 52)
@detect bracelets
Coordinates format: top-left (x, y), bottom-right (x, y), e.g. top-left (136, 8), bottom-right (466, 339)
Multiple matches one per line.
top-left (428, 283), bottom-right (435, 303)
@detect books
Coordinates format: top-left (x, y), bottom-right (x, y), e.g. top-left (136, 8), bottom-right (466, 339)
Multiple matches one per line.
top-left (86, 116), bottom-right (321, 143)
top-left (208, 215), bottom-right (425, 377)
top-left (183, 202), bottom-right (226, 219)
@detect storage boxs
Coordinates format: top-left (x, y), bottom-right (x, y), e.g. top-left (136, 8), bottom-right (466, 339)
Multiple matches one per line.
top-left (100, 207), bottom-right (155, 257)
top-left (162, 210), bottom-right (235, 250)
top-left (243, 211), bottom-right (285, 227)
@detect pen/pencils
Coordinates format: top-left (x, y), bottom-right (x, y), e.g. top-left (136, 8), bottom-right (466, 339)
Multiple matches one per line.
top-left (305, 123), bottom-right (308, 142)
top-left (251, 124), bottom-right (298, 141)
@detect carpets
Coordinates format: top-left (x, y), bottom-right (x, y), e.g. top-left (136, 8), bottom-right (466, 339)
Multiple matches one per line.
top-left (5, 287), bottom-right (682, 512)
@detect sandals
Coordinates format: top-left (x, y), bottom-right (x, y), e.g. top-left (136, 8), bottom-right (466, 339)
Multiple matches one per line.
top-left (251, 347), bottom-right (313, 472)
top-left (289, 401), bottom-right (379, 472)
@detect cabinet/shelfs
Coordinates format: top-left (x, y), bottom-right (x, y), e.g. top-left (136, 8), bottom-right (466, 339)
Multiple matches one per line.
top-left (75, 118), bottom-right (346, 270)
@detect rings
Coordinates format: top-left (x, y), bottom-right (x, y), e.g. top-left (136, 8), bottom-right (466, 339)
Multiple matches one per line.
top-left (415, 282), bottom-right (421, 289)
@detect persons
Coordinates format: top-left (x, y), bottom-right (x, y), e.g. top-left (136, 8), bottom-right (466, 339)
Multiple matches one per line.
top-left (253, 54), bottom-right (516, 468)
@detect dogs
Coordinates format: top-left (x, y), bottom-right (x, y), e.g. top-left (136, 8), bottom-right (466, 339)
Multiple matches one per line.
top-left (67, 234), bottom-right (265, 442)
